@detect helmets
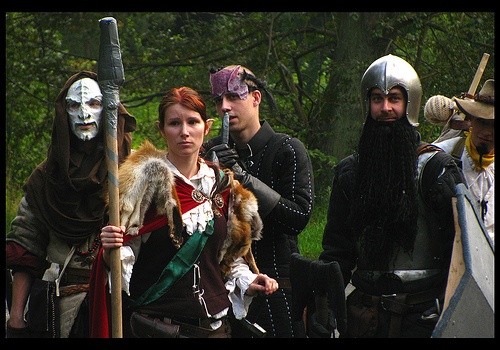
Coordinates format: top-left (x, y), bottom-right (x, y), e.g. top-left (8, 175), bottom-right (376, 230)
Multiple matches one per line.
top-left (361, 55), bottom-right (423, 128)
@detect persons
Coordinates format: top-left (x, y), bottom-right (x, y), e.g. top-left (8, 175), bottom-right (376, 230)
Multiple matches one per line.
top-left (200, 65), bottom-right (314, 337)
top-left (303, 54), bottom-right (469, 338)
top-left (99, 86), bottom-right (280, 337)
top-left (6, 70), bottom-right (135, 338)
top-left (430, 78), bottom-right (494, 251)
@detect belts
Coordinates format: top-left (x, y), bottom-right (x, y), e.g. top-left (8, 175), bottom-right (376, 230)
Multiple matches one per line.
top-left (354, 292), bottom-right (443, 315)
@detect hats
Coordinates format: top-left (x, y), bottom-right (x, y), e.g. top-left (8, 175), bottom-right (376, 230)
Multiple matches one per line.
top-left (453, 79), bottom-right (494, 120)
